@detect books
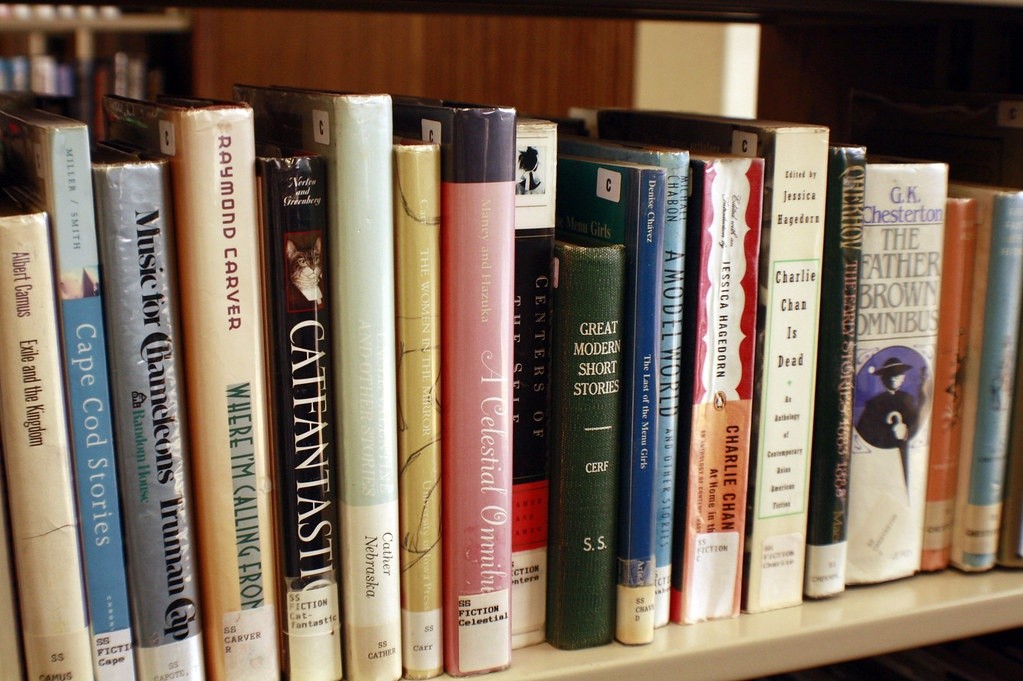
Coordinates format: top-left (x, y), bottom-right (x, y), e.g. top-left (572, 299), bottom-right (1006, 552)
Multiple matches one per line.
top-left (0, 0), bottom-right (164, 143)
top-left (0, 86), bottom-right (1023, 681)
